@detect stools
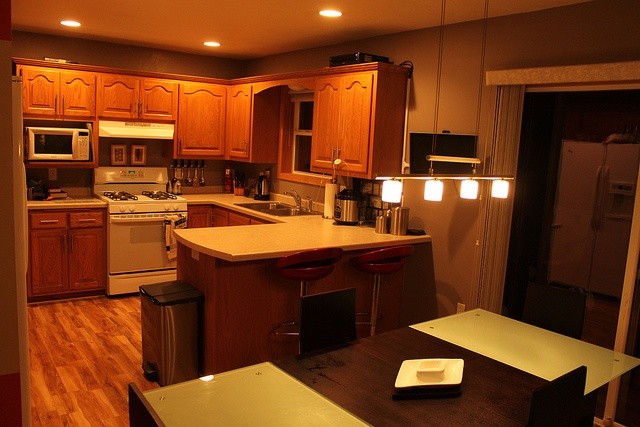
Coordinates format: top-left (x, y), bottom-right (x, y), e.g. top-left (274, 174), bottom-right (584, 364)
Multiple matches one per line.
top-left (357, 244), bottom-right (414, 336)
top-left (271, 246), bottom-right (344, 356)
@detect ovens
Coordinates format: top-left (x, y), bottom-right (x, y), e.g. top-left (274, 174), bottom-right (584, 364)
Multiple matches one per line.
top-left (91, 167), bottom-right (188, 298)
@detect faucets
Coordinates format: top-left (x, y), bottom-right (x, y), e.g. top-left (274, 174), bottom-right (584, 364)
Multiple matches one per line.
top-left (283, 191), bottom-right (301, 208)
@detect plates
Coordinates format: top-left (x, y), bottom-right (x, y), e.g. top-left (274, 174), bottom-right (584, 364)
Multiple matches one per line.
top-left (394, 358), bottom-right (464, 389)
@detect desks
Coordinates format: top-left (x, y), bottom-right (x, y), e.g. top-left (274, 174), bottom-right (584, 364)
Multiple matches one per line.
top-left (172, 189), bottom-right (432, 376)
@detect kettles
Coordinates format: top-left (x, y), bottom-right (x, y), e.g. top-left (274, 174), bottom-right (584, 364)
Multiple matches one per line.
top-left (253, 175), bottom-right (270, 200)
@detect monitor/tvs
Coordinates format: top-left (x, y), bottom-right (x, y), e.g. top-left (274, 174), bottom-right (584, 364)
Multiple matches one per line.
top-left (409, 133), bottom-right (477, 174)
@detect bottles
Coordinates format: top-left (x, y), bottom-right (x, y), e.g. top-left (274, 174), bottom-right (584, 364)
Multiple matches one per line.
top-left (375, 216), bottom-right (388, 234)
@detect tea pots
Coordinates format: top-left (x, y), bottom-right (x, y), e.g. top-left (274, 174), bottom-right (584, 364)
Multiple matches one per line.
top-left (390, 206), bottom-right (410, 236)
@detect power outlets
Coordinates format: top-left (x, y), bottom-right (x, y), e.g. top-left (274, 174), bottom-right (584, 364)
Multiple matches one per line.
top-left (455, 302), bottom-right (466, 313)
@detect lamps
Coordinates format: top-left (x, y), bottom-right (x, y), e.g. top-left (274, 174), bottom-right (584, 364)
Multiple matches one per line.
top-left (491, 178), bottom-right (509, 199)
top-left (459, 179), bottom-right (479, 202)
top-left (380, 177), bottom-right (403, 204)
top-left (424, 177), bottom-right (445, 203)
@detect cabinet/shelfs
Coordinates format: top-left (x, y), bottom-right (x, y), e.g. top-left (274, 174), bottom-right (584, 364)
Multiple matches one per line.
top-left (227, 83), bottom-right (253, 160)
top-left (180, 83), bottom-right (226, 155)
top-left (27, 210), bottom-right (108, 296)
top-left (22, 67), bottom-right (96, 116)
top-left (97, 74), bottom-right (179, 119)
top-left (188, 208), bottom-right (212, 229)
top-left (311, 75), bottom-right (369, 175)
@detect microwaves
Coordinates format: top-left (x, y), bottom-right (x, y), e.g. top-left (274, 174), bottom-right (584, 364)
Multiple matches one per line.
top-left (24, 126), bottom-right (90, 161)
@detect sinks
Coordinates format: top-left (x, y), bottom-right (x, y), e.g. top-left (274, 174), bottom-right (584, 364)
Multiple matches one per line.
top-left (233, 201), bottom-right (304, 208)
top-left (252, 208), bottom-right (324, 217)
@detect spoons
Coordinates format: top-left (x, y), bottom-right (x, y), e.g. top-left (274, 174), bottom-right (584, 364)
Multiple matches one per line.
top-left (186, 159), bottom-right (192, 186)
top-left (200, 159), bottom-right (205, 185)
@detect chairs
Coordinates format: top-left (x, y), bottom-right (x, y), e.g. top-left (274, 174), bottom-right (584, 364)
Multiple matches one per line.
top-left (297, 289), bottom-right (359, 353)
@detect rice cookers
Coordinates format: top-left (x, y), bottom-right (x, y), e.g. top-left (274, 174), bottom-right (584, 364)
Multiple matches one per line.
top-left (334, 189), bottom-right (371, 224)
